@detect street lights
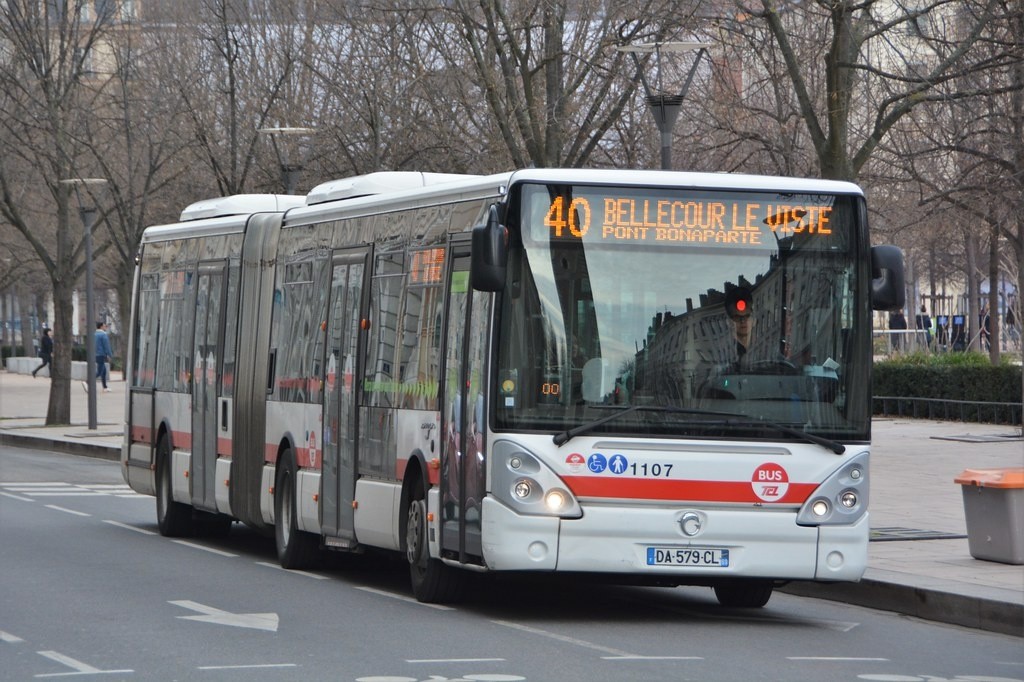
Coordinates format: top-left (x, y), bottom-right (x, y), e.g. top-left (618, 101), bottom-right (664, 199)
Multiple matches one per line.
top-left (256, 128), bottom-right (318, 194)
top-left (61, 179), bottom-right (109, 431)
top-left (616, 42), bottom-right (717, 171)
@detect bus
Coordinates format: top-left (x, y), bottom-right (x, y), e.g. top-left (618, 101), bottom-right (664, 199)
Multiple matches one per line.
top-left (122, 166), bottom-right (906, 609)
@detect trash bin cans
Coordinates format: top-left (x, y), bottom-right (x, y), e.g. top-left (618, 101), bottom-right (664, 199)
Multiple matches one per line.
top-left (954, 468), bottom-right (1024, 565)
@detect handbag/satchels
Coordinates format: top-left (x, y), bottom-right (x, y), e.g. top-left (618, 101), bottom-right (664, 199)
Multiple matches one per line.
top-left (38, 347), bottom-right (42, 357)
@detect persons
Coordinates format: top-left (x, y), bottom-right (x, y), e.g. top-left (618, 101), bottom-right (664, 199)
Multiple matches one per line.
top-left (888, 308), bottom-right (908, 353)
top-left (979, 309), bottom-right (991, 352)
top-left (82, 321), bottom-right (113, 392)
top-left (691, 298), bottom-right (799, 400)
top-left (31, 328), bottom-right (54, 378)
top-left (917, 304), bottom-right (932, 351)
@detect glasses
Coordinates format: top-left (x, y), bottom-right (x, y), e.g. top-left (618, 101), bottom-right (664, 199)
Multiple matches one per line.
top-left (728, 314), bottom-right (752, 322)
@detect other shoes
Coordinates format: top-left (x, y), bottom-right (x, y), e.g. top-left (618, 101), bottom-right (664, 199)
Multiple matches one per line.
top-left (103, 387), bottom-right (111, 392)
top-left (82, 381), bottom-right (89, 393)
top-left (32, 371), bottom-right (35, 377)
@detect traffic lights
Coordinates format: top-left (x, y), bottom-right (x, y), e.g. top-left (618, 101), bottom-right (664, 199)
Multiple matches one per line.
top-left (724, 275), bottom-right (753, 319)
